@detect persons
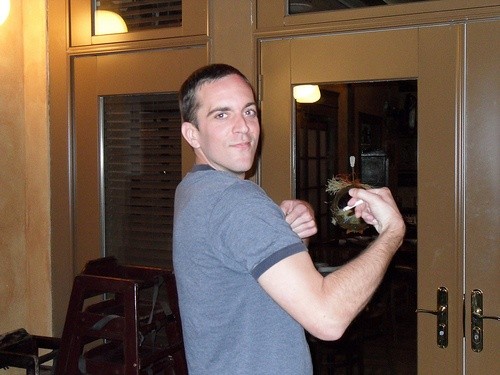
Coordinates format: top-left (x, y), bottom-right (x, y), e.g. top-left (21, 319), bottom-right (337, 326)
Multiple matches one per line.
top-left (170, 64), bottom-right (405, 375)
